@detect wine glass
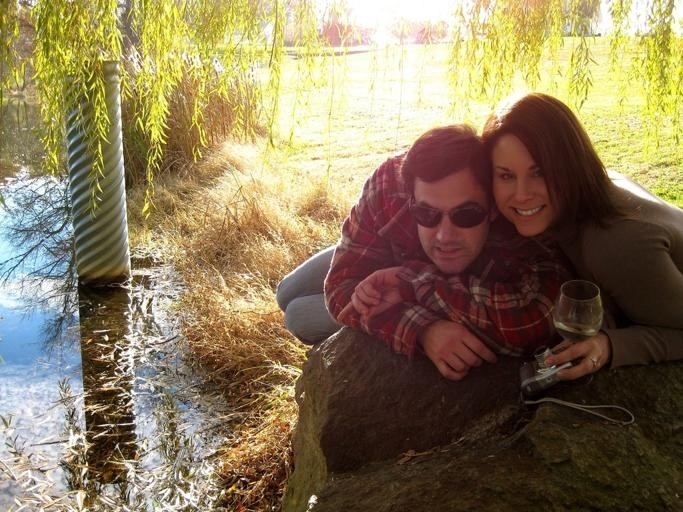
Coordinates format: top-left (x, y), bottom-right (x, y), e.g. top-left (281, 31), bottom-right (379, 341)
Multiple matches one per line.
top-left (552, 280), bottom-right (604, 388)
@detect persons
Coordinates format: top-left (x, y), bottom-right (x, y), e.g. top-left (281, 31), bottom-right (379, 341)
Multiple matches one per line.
top-left (482, 90), bottom-right (683, 383)
top-left (273, 124), bottom-right (579, 381)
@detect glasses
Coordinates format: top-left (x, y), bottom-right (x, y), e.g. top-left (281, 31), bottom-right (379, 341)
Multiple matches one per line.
top-left (407, 196), bottom-right (494, 230)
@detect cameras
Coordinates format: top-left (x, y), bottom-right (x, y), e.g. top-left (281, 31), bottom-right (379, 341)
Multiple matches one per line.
top-left (519, 344), bottom-right (573, 393)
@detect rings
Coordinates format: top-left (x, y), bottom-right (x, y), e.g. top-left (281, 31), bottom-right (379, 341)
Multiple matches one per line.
top-left (595, 361), bottom-right (600, 367)
top-left (587, 354), bottom-right (599, 369)
